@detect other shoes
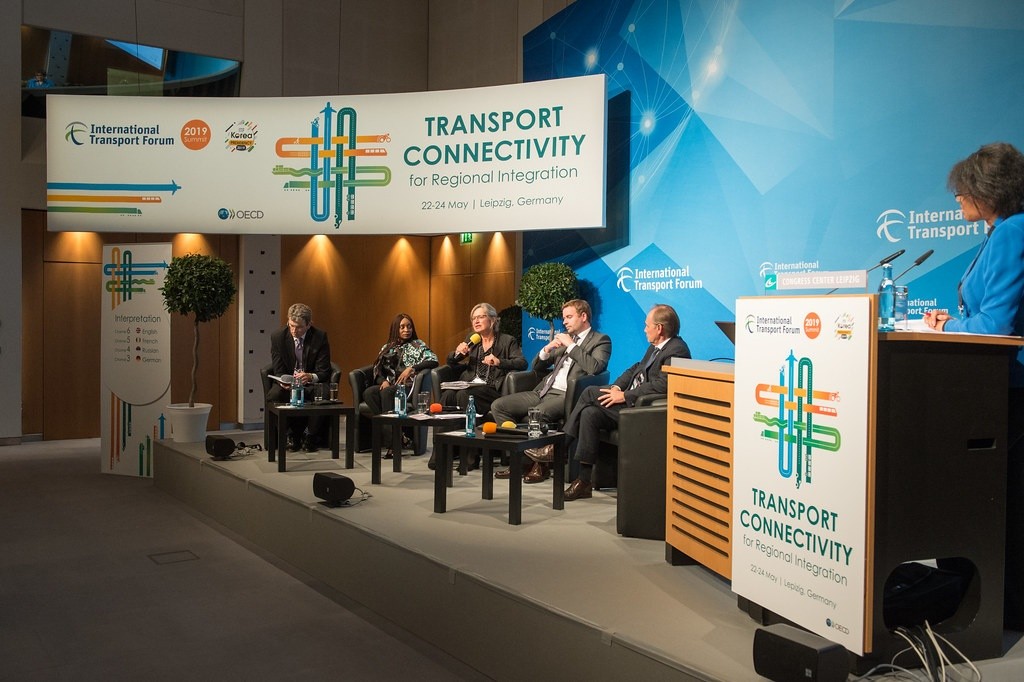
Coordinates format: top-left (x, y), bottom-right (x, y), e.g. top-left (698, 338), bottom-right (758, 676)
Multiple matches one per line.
top-left (383, 448), bottom-right (392, 459)
top-left (302, 435), bottom-right (316, 451)
top-left (402, 432), bottom-right (411, 449)
top-left (285, 437), bottom-right (302, 450)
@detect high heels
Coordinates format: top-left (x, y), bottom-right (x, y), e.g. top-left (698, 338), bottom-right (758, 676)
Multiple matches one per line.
top-left (428, 454), bottom-right (435, 470)
top-left (456, 456), bottom-right (481, 472)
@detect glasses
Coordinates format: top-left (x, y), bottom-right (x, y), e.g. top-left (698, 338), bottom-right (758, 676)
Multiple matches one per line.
top-left (953, 191), bottom-right (971, 202)
top-left (471, 315), bottom-right (487, 323)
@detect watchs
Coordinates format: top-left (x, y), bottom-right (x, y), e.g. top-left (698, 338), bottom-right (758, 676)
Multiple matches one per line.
top-left (310, 374), bottom-right (314, 382)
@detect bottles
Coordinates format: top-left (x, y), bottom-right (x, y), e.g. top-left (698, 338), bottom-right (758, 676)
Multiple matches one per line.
top-left (466, 396), bottom-right (476, 437)
top-left (395, 384), bottom-right (402, 414)
top-left (290, 378), bottom-right (297, 406)
top-left (296, 378), bottom-right (304, 406)
top-left (399, 385), bottom-right (407, 417)
top-left (877, 262), bottom-right (896, 333)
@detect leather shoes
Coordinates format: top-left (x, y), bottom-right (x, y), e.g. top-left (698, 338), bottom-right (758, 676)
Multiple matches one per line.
top-left (563, 480), bottom-right (593, 501)
top-left (523, 462), bottom-right (551, 483)
top-left (494, 466), bottom-right (511, 479)
top-left (524, 444), bottom-right (553, 463)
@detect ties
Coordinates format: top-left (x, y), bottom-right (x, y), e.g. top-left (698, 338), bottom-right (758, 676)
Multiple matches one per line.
top-left (295, 337), bottom-right (303, 373)
top-left (642, 347), bottom-right (660, 383)
top-left (539, 335), bottom-right (580, 398)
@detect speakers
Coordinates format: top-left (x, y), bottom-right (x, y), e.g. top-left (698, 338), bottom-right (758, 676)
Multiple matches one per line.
top-left (206, 435), bottom-right (235, 457)
top-left (314, 472), bottom-right (356, 503)
top-left (751, 624), bottom-right (851, 682)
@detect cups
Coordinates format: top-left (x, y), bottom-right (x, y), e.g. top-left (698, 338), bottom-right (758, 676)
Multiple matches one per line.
top-left (418, 391), bottom-right (430, 417)
top-left (313, 383), bottom-right (323, 401)
top-left (896, 287), bottom-right (908, 331)
top-left (528, 407), bottom-right (540, 437)
top-left (473, 424), bottom-right (487, 439)
top-left (330, 383), bottom-right (338, 401)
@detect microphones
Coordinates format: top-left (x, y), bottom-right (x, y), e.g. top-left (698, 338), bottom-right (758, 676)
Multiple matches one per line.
top-left (482, 421), bottom-right (529, 436)
top-left (893, 249), bottom-right (935, 281)
top-left (454, 334), bottom-right (481, 361)
top-left (281, 374), bottom-right (294, 382)
top-left (825, 249), bottom-right (906, 295)
top-left (429, 403), bottom-right (463, 413)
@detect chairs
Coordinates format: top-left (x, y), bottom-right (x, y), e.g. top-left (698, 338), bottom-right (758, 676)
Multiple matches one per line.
top-left (259, 360), bottom-right (342, 450)
top-left (506, 367), bottom-right (610, 471)
top-left (616, 399), bottom-right (669, 542)
top-left (430, 361), bottom-right (514, 458)
top-left (590, 391), bottom-right (667, 492)
top-left (349, 366), bottom-right (432, 456)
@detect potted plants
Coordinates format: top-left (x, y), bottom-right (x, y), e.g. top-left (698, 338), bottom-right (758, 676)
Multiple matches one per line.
top-left (156, 254), bottom-right (239, 443)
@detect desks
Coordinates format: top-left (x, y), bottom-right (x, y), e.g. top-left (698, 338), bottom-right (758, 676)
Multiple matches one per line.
top-left (433, 430), bottom-right (566, 525)
top-left (266, 402), bottom-right (355, 472)
top-left (371, 414), bottom-right (486, 486)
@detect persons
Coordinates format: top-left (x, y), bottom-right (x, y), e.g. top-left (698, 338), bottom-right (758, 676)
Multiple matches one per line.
top-left (922, 142), bottom-right (1024, 607)
top-left (264, 303), bottom-right (331, 453)
top-left (26, 68), bottom-right (54, 87)
top-left (427, 303), bottom-right (529, 473)
top-left (524, 304), bottom-right (691, 501)
top-left (491, 298), bottom-right (611, 484)
top-left (363, 312), bottom-right (440, 460)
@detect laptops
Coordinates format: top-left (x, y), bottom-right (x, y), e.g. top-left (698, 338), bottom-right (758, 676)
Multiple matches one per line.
top-left (715, 321), bottom-right (735, 345)
